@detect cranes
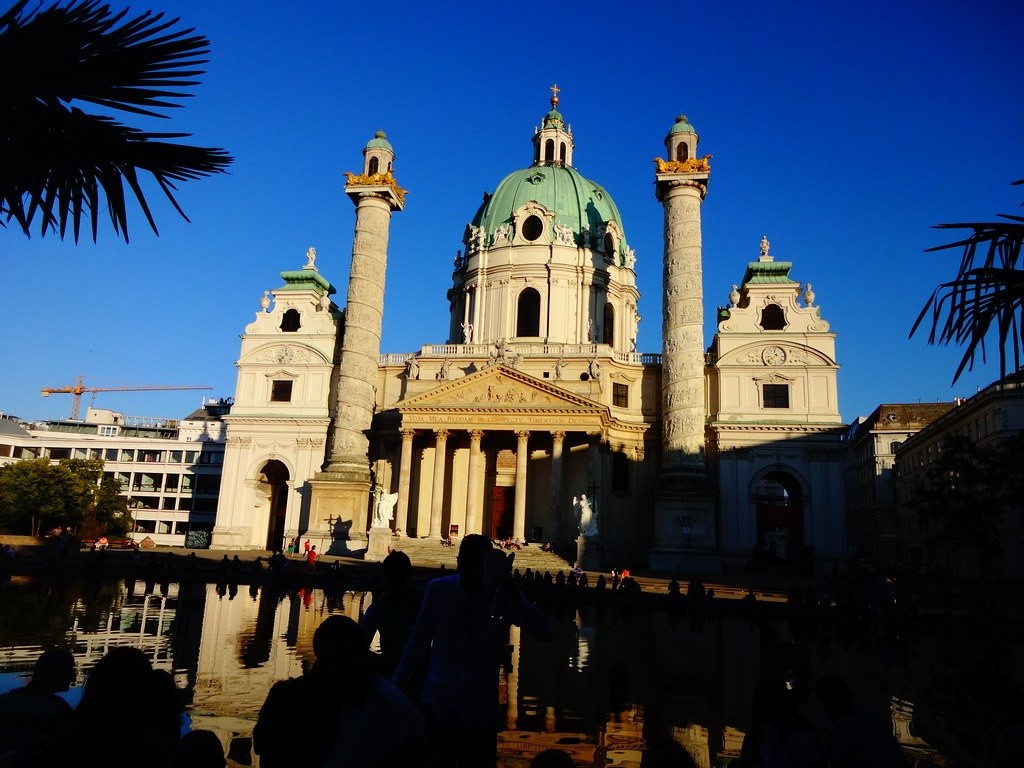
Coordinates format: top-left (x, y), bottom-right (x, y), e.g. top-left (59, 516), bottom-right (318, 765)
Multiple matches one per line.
top-left (40, 374), bottom-right (214, 420)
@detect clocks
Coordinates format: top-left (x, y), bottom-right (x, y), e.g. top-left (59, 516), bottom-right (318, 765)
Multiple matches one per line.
top-left (765, 347), bottom-right (786, 366)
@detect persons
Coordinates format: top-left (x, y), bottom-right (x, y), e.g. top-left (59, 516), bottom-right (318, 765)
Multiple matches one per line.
top-left (253, 527), bottom-right (1024, 768)
top-left (0, 643), bottom-right (228, 768)
top-left (378, 489), bottom-right (387, 525)
top-left (579, 494), bottom-right (593, 532)
top-left (677, 514), bottom-right (696, 546)
top-left (0, 543), bottom-right (15, 559)
top-left (50, 526), bottom-right (73, 537)
top-left (93, 536), bottom-right (109, 551)
